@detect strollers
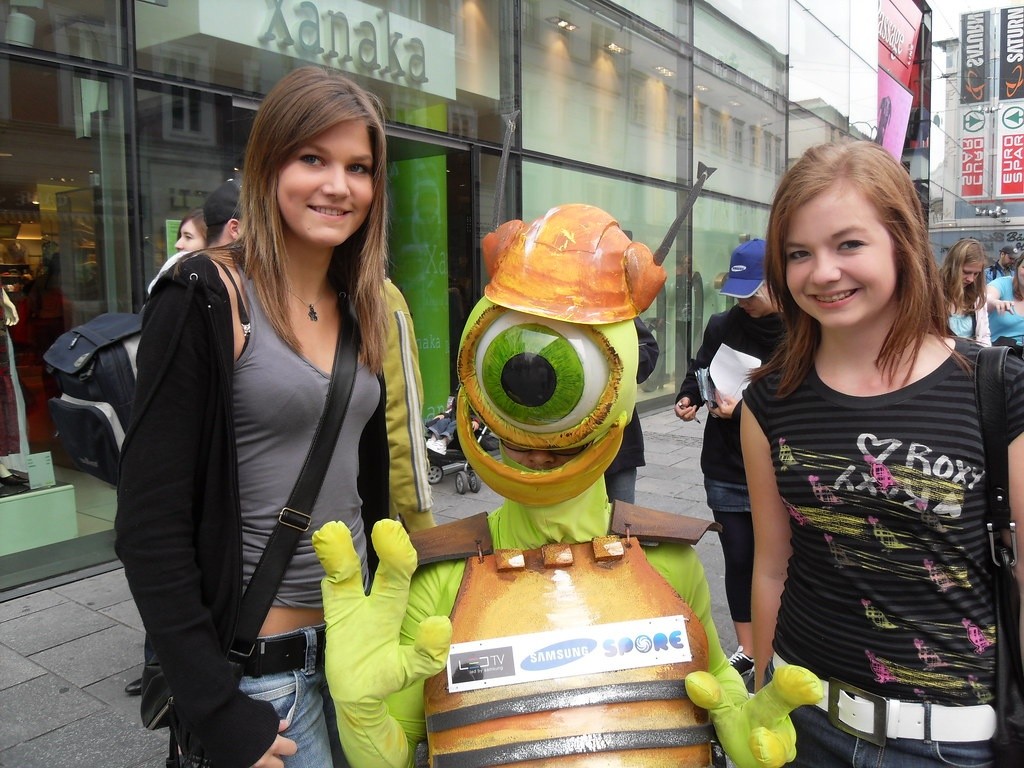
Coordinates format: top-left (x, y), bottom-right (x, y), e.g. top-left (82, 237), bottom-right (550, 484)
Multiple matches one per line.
top-left (423, 383), bottom-right (499, 496)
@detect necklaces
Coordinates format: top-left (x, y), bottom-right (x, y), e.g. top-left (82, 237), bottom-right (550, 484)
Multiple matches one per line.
top-left (285, 287), bottom-right (323, 322)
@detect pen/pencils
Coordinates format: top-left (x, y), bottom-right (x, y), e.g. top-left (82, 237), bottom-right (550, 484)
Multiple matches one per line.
top-left (678, 401), bottom-right (702, 424)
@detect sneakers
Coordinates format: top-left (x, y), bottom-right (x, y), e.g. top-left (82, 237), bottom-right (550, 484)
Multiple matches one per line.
top-left (727, 645), bottom-right (755, 676)
top-left (430, 439), bottom-right (447, 455)
top-left (425, 437), bottom-right (436, 448)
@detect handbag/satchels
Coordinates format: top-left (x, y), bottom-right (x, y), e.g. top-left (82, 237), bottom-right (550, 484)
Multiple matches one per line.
top-left (974, 345), bottom-right (1024, 768)
top-left (141, 627), bottom-right (183, 731)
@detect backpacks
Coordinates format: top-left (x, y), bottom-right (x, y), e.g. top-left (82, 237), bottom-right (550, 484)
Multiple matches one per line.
top-left (42, 310), bottom-right (144, 486)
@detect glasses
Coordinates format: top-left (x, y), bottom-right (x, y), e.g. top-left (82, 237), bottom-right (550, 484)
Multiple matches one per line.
top-left (500, 439), bottom-right (593, 456)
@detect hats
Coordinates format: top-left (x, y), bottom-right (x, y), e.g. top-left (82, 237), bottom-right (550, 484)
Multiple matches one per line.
top-left (1001, 245), bottom-right (1018, 258)
top-left (719, 238), bottom-right (769, 298)
top-left (203, 177), bottom-right (246, 227)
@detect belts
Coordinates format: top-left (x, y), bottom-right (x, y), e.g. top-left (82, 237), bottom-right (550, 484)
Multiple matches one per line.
top-left (772, 649), bottom-right (995, 746)
top-left (242, 628), bottom-right (328, 677)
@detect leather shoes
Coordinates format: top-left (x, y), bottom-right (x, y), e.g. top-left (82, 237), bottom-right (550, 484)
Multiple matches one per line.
top-left (0, 474), bottom-right (29, 485)
top-left (125, 678), bottom-right (141, 695)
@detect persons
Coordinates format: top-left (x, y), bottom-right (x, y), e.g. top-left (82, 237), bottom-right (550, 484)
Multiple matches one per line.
top-left (984, 246), bottom-right (1019, 285)
top-left (114, 69), bottom-right (397, 768)
top-left (603, 317), bottom-right (660, 503)
top-left (675, 238), bottom-right (791, 681)
top-left (985, 252), bottom-right (1024, 346)
top-left (147, 177), bottom-right (246, 311)
top-left (312, 202), bottom-right (823, 768)
top-left (375, 278), bottom-right (438, 534)
top-left (938, 239), bottom-right (992, 347)
top-left (740, 141), bottom-right (1024, 767)
top-left (175, 209), bottom-right (208, 255)
